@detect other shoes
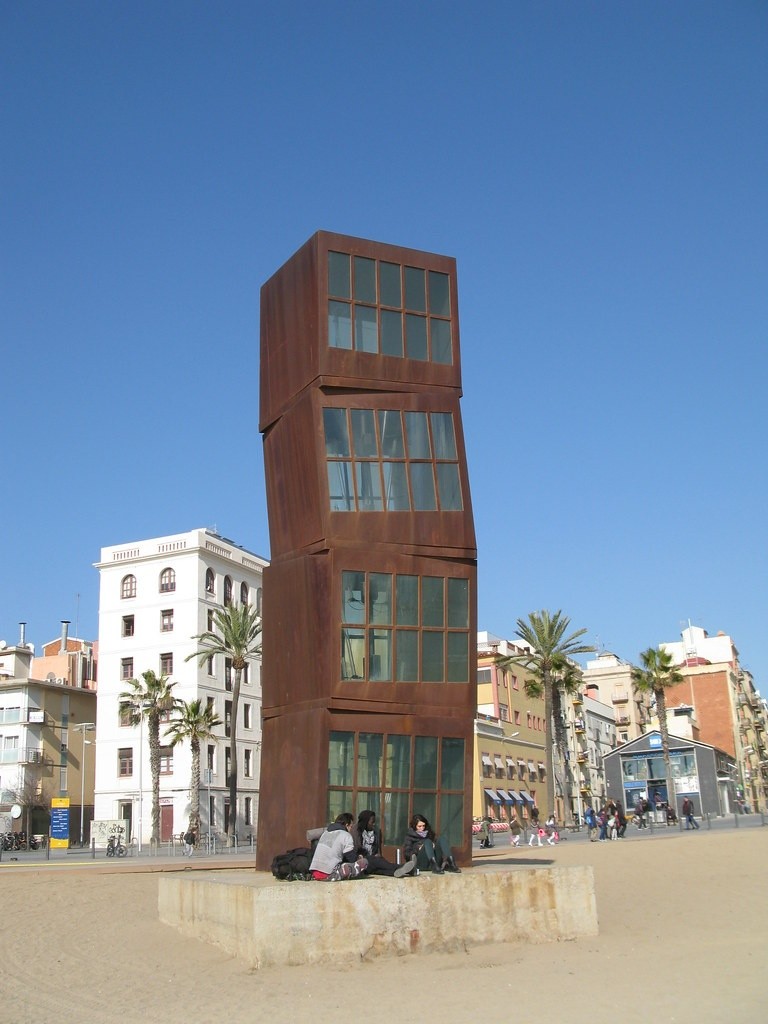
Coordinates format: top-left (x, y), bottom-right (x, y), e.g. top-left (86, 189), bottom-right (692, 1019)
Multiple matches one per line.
top-left (538, 844), bottom-right (543, 846)
top-left (407, 853), bottom-right (417, 876)
top-left (529, 843), bottom-right (532, 847)
top-left (591, 839), bottom-right (596, 842)
top-left (481, 846), bottom-right (485, 849)
top-left (394, 861), bottom-right (415, 877)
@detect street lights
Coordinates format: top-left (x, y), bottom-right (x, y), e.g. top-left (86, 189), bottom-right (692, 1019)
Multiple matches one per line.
top-left (72, 723), bottom-right (95, 848)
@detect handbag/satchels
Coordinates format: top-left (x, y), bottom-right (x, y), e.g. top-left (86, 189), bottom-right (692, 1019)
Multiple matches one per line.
top-left (476, 831), bottom-right (486, 840)
top-left (607, 817), bottom-right (615, 827)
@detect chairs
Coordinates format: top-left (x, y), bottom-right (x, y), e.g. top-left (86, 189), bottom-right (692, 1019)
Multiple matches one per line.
top-left (175, 832), bottom-right (184, 843)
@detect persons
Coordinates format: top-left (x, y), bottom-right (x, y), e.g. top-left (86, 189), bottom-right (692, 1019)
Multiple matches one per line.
top-left (584, 798), bottom-right (627, 842)
top-left (402, 814), bottom-right (461, 874)
top-left (682, 796), bottom-right (700, 830)
top-left (636, 799), bottom-right (648, 830)
top-left (308, 813), bottom-right (368, 882)
top-left (532, 806), bottom-right (539, 820)
top-left (666, 807), bottom-right (677, 826)
top-left (182, 829), bottom-right (196, 856)
top-left (544, 815), bottom-right (556, 845)
top-left (509, 815), bottom-right (524, 847)
top-left (528, 819), bottom-right (543, 846)
top-left (352, 810), bottom-right (418, 877)
top-left (479, 817), bottom-right (495, 849)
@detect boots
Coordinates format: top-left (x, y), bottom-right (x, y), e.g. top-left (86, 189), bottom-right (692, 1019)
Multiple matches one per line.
top-left (431, 857), bottom-right (444, 874)
top-left (447, 855), bottom-right (461, 873)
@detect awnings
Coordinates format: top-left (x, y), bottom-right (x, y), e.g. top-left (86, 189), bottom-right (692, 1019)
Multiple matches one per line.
top-left (520, 791), bottom-right (534, 804)
top-left (497, 790), bottom-right (513, 805)
top-left (508, 791), bottom-right (524, 805)
top-left (485, 789), bottom-right (502, 805)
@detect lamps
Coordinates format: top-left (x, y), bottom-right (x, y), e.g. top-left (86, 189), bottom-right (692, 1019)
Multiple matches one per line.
top-left (583, 750), bottom-right (590, 754)
top-left (84, 741), bottom-right (96, 747)
top-left (501, 732), bottom-right (520, 745)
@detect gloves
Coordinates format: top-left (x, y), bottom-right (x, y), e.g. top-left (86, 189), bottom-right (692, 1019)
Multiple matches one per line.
top-left (357, 847), bottom-right (367, 857)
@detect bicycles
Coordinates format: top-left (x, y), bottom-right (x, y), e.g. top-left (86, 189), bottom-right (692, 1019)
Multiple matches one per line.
top-left (0, 832), bottom-right (39, 850)
top-left (106, 835), bottom-right (128, 858)
